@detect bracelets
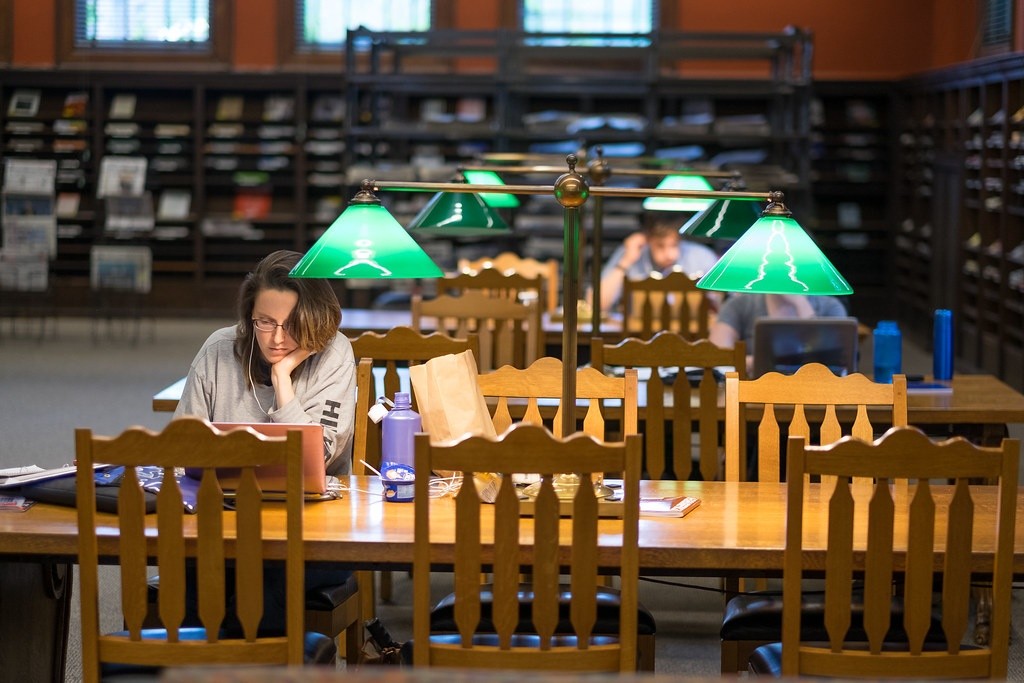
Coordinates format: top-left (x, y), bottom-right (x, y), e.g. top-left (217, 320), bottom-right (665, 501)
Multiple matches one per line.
top-left (616, 263), bottom-right (629, 273)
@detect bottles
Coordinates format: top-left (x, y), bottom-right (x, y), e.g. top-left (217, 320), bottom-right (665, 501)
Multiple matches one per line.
top-left (381, 391), bottom-right (421, 469)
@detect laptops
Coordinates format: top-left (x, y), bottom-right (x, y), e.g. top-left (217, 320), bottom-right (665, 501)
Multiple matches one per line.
top-left (207, 417), bottom-right (338, 501)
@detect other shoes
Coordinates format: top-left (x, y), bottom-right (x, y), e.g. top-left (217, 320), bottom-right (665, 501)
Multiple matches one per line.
top-left (303, 632), bottom-right (338, 668)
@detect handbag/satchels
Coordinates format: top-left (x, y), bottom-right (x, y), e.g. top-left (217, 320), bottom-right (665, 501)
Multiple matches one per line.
top-left (355, 616), bottom-right (415, 677)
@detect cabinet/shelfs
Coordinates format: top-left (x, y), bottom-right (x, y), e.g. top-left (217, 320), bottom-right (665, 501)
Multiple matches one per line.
top-left (0, 50), bottom-right (1024, 388)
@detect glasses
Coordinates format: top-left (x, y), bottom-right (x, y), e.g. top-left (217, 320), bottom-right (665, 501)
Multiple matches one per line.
top-left (251, 314), bottom-right (295, 335)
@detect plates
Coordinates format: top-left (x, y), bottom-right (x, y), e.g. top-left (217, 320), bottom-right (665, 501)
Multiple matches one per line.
top-left (525, 482), bottom-right (615, 503)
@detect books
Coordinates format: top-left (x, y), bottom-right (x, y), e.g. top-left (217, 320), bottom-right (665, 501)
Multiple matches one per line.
top-left (0, 495), bottom-right (37, 512)
top-left (639, 497), bottom-right (701, 517)
top-left (0, 464), bottom-right (110, 487)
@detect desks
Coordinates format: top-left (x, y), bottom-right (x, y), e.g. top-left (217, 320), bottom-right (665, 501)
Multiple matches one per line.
top-left (0, 472), bottom-right (1024, 683)
top-left (484, 374), bottom-right (1024, 483)
top-left (332, 307), bottom-right (870, 366)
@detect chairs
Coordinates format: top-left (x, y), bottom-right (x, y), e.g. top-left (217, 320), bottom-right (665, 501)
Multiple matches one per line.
top-left (72, 248), bottom-right (1024, 683)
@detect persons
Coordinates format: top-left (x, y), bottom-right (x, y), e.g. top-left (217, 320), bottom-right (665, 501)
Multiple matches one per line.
top-left (173, 250), bottom-right (357, 640)
top-left (585, 210), bottom-right (725, 316)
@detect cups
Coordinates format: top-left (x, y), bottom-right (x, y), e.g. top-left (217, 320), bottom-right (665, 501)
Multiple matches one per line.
top-left (872, 321), bottom-right (903, 383)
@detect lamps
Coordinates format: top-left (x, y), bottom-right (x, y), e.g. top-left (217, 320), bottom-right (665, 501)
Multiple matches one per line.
top-left (287, 140), bottom-right (855, 440)
